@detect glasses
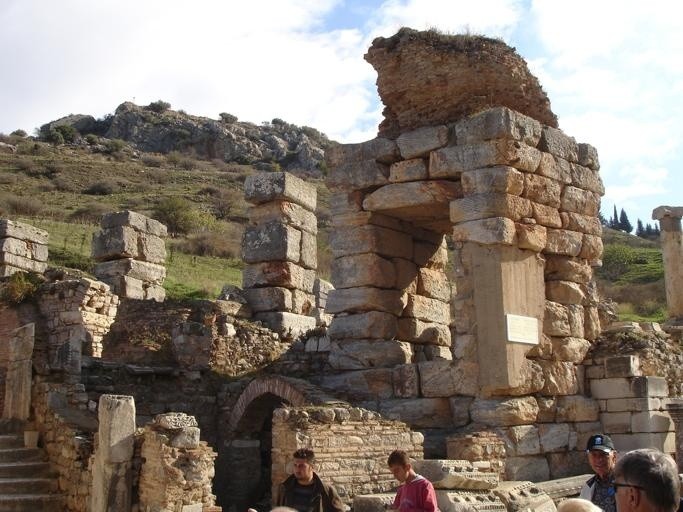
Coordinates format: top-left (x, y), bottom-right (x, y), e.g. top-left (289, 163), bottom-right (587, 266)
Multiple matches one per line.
top-left (610, 478), bottom-right (644, 495)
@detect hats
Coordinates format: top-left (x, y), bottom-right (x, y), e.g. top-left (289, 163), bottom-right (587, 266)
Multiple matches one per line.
top-left (585, 432), bottom-right (614, 457)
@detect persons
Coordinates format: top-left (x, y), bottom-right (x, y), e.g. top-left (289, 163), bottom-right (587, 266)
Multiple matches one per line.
top-left (388, 450), bottom-right (438, 512)
top-left (557, 434), bottom-right (683, 512)
top-left (276, 448), bottom-right (346, 512)
